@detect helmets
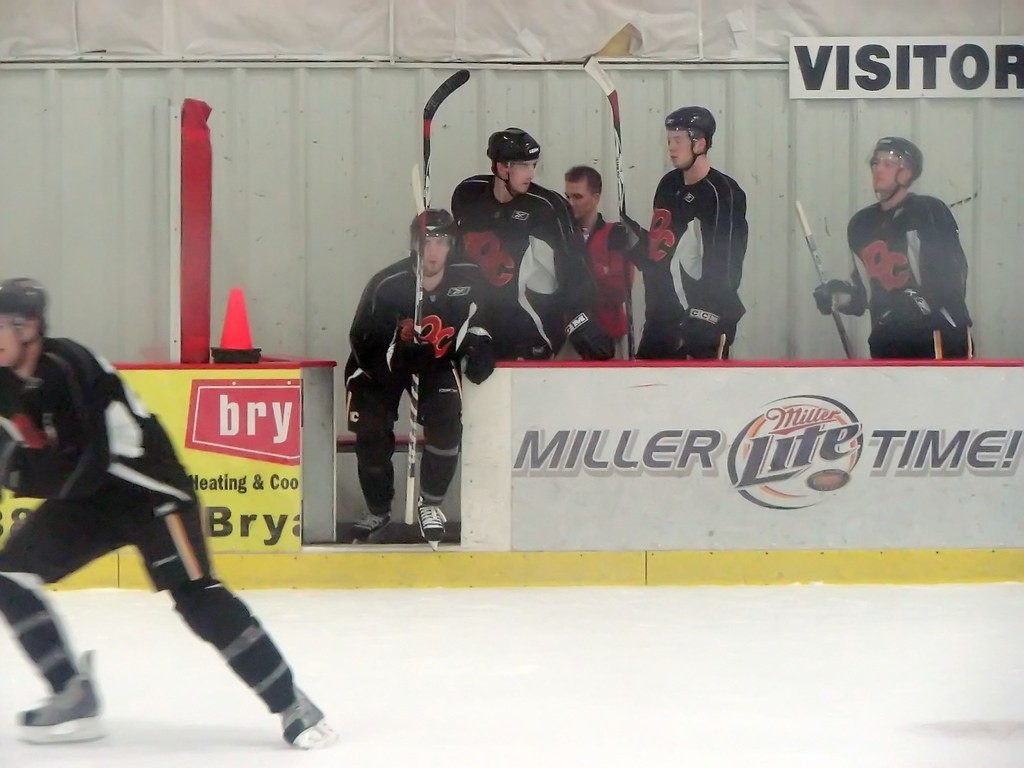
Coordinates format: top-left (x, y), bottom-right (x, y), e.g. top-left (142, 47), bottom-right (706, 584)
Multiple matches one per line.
top-left (486, 127), bottom-right (541, 160)
top-left (664, 108), bottom-right (718, 137)
top-left (410, 210), bottom-right (458, 242)
top-left (0, 279), bottom-right (47, 314)
top-left (875, 137), bottom-right (923, 182)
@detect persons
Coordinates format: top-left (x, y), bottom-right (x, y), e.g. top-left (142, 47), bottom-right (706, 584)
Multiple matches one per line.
top-left (0, 277), bottom-right (338, 751)
top-left (343, 208), bottom-right (493, 554)
top-left (451, 127), bottom-right (615, 362)
top-left (607, 107), bottom-right (748, 360)
top-left (813, 137), bottom-right (976, 360)
top-left (549, 166), bottom-right (635, 361)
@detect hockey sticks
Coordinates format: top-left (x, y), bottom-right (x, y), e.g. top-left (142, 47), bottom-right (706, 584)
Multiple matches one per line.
top-left (794, 199), bottom-right (855, 359)
top-left (582, 55), bottom-right (635, 360)
top-left (423, 69), bottom-right (470, 210)
top-left (405, 164), bottom-right (431, 525)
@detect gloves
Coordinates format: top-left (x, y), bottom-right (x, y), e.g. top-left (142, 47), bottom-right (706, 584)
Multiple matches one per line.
top-left (683, 318), bottom-right (723, 361)
top-left (813, 279), bottom-right (866, 317)
top-left (386, 342), bottom-right (434, 377)
top-left (566, 314), bottom-right (617, 362)
top-left (458, 333), bottom-right (497, 385)
top-left (873, 285), bottom-right (934, 331)
top-left (608, 217), bottom-right (651, 272)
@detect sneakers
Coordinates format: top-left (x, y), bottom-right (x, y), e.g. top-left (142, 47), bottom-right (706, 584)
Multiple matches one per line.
top-left (278, 684), bottom-right (337, 749)
top-left (14, 649), bottom-right (105, 742)
top-left (351, 507), bottom-right (391, 545)
top-left (416, 495), bottom-right (447, 551)
